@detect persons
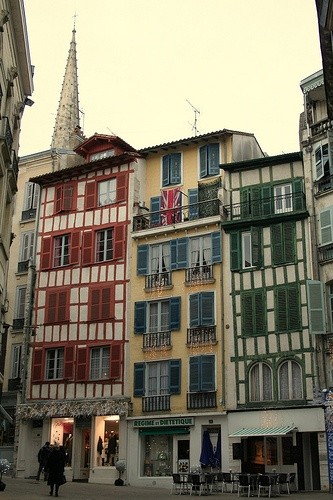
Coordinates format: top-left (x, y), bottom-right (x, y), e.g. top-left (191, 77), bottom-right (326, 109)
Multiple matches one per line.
top-left (36, 441), bottom-right (50, 481)
top-left (45, 440), bottom-right (67, 497)
top-left (64, 433), bottom-right (72, 467)
top-left (105, 430), bottom-right (117, 466)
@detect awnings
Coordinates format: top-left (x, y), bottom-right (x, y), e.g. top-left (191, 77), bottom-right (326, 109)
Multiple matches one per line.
top-left (229, 426), bottom-right (297, 437)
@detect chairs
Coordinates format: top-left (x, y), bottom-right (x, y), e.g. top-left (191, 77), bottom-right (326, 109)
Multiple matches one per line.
top-left (170, 473), bottom-right (297, 500)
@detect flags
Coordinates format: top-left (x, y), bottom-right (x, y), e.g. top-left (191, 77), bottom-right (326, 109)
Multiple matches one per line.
top-left (159, 187), bottom-right (181, 226)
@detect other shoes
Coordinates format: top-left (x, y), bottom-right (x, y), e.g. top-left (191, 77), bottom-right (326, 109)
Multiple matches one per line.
top-left (55, 494), bottom-right (58, 497)
top-left (44, 479), bottom-right (47, 481)
top-left (35, 477), bottom-right (40, 481)
top-left (49, 492), bottom-right (54, 495)
